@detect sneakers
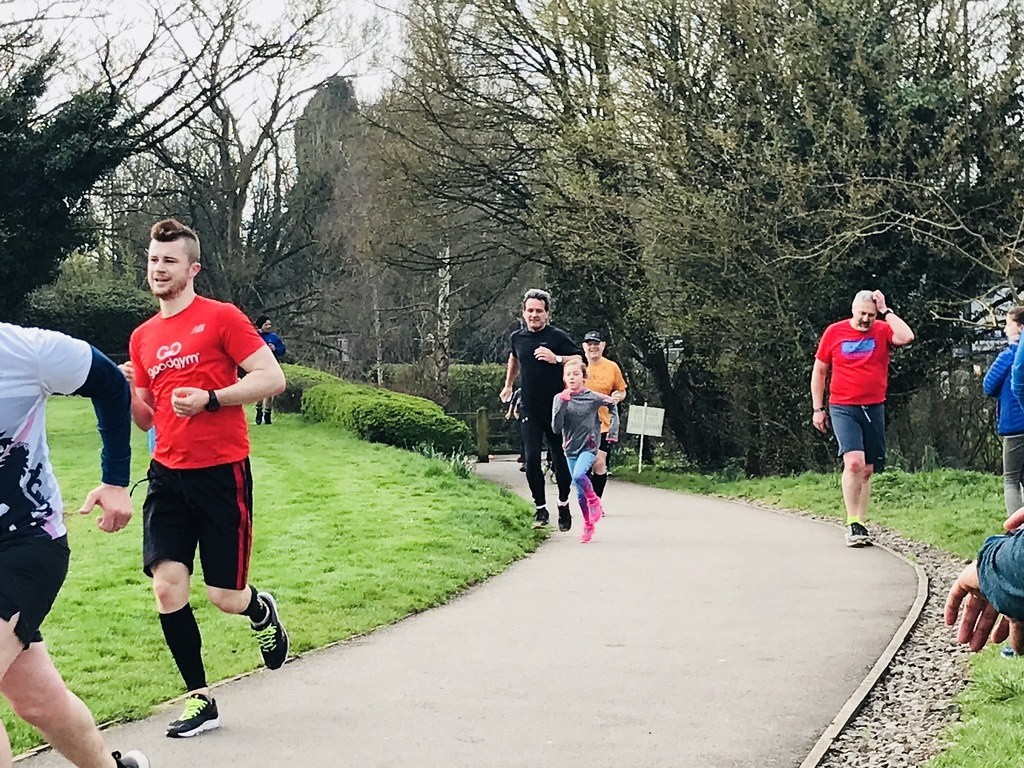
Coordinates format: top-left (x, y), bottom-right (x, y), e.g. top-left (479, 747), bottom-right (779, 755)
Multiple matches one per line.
top-left (588, 497), bottom-right (602, 524)
top-left (533, 507), bottom-right (550, 529)
top-left (580, 524), bottom-right (595, 543)
top-left (845, 522), bottom-right (873, 548)
top-left (165, 693), bottom-right (220, 738)
top-left (250, 592), bottom-right (289, 670)
top-left (558, 503), bottom-right (572, 532)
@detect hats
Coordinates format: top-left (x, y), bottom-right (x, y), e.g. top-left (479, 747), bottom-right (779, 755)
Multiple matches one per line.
top-left (583, 331), bottom-right (603, 342)
top-left (256, 315), bottom-right (270, 329)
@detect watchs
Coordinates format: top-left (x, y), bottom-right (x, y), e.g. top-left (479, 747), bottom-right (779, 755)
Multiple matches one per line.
top-left (882, 308), bottom-right (893, 319)
top-left (204, 389), bottom-right (221, 413)
top-left (556, 355), bottom-right (563, 364)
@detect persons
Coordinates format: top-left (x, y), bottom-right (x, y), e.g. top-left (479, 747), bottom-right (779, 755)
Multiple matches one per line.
top-left (582, 330), bottom-right (627, 518)
top-left (499, 289), bottom-right (582, 532)
top-left (115, 218), bottom-right (290, 738)
top-left (0, 322), bottom-right (151, 768)
top-left (252, 316), bottom-right (286, 425)
top-left (605, 405), bottom-right (620, 476)
top-left (504, 388), bottom-right (555, 477)
top-left (551, 359), bottom-right (620, 543)
top-left (811, 289), bottom-right (915, 547)
top-left (983, 306), bottom-right (1024, 659)
top-left (944, 505), bottom-right (1024, 657)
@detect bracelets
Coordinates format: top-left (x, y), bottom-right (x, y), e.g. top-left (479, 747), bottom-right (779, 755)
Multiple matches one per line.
top-left (814, 406), bottom-right (825, 412)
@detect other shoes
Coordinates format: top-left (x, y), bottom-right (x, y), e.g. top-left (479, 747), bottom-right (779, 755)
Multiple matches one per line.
top-left (111, 750), bottom-right (150, 768)
top-left (255, 412), bottom-right (263, 425)
top-left (1001, 646), bottom-right (1017, 659)
top-left (601, 511), bottom-right (605, 517)
top-left (264, 415), bottom-right (271, 424)
top-left (520, 467), bottom-right (526, 472)
top-left (549, 472), bottom-right (557, 484)
top-left (541, 460), bottom-right (550, 476)
top-left (516, 456), bottom-right (523, 463)
